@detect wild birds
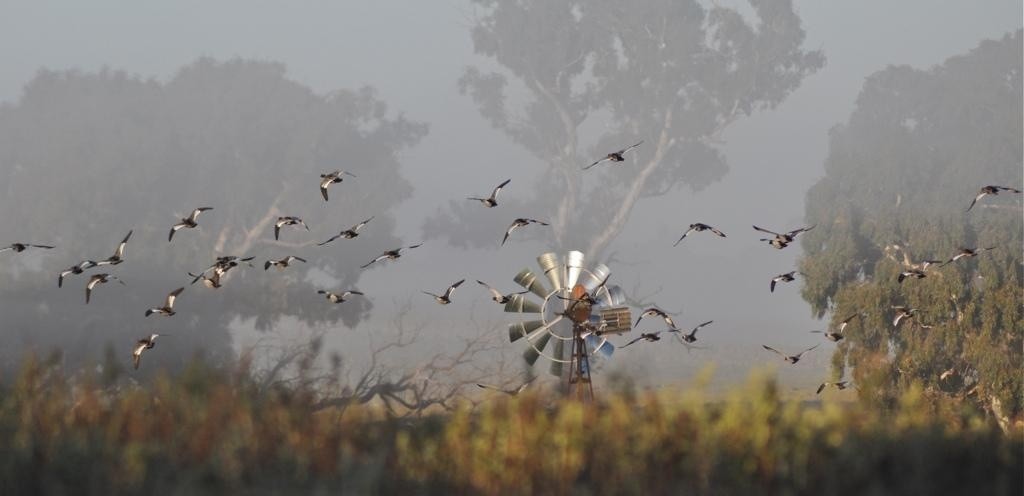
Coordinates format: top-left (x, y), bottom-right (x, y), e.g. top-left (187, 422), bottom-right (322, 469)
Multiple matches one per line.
top-left (169, 208), bottom-right (213, 243)
top-left (264, 256), bottom-right (307, 271)
top-left (358, 243), bottom-right (423, 269)
top-left (752, 221), bottom-right (817, 250)
top-left (130, 331), bottom-right (162, 371)
top-left (317, 289), bottom-right (366, 304)
top-left (891, 307), bottom-right (930, 328)
top-left (675, 222), bottom-right (727, 247)
top-left (897, 244), bottom-right (996, 284)
top-left (769, 269), bottom-right (812, 292)
top-left (475, 279), bottom-right (529, 305)
top-left (420, 280), bottom-right (464, 306)
top-left (85, 229), bottom-right (133, 271)
top-left (188, 255), bottom-right (255, 289)
top-left (467, 177), bottom-right (549, 246)
top-left (966, 183), bottom-right (1020, 210)
top-left (319, 172), bottom-right (351, 201)
top-left (86, 272), bottom-right (126, 305)
top-left (317, 215), bottom-right (375, 247)
top-left (60, 259), bottom-right (93, 288)
top-left (619, 307), bottom-right (713, 350)
top-left (583, 138), bottom-right (647, 173)
top-left (274, 216), bottom-right (309, 241)
top-left (817, 378), bottom-right (854, 394)
top-left (0, 241), bottom-right (57, 254)
top-left (762, 313), bottom-right (859, 365)
